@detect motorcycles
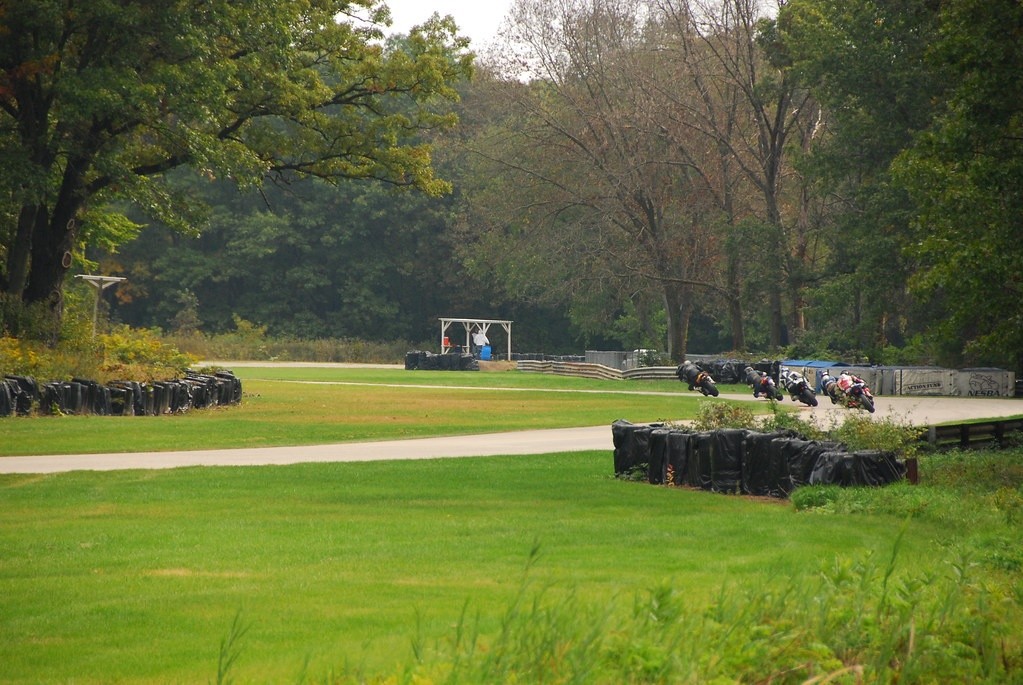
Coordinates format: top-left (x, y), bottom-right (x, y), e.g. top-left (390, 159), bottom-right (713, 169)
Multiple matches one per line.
top-left (825, 379), bottom-right (875, 413)
top-left (693, 370), bottom-right (719, 397)
top-left (760, 376), bottom-right (783, 402)
top-left (783, 377), bottom-right (818, 406)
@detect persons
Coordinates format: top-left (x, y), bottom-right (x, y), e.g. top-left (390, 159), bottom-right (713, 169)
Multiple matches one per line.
top-left (743, 366), bottom-right (874, 410)
top-left (471, 328), bottom-right (485, 360)
top-left (684, 360), bottom-right (716, 391)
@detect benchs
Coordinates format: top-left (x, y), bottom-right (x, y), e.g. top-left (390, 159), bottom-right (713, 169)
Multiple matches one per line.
top-left (441, 345), bottom-right (470, 355)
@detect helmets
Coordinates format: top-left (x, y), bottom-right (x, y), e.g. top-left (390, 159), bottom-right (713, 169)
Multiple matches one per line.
top-left (841, 370), bottom-right (849, 375)
top-left (820, 372), bottom-right (829, 378)
top-left (744, 367), bottom-right (753, 374)
top-left (684, 360), bottom-right (691, 366)
top-left (781, 368), bottom-right (789, 373)
